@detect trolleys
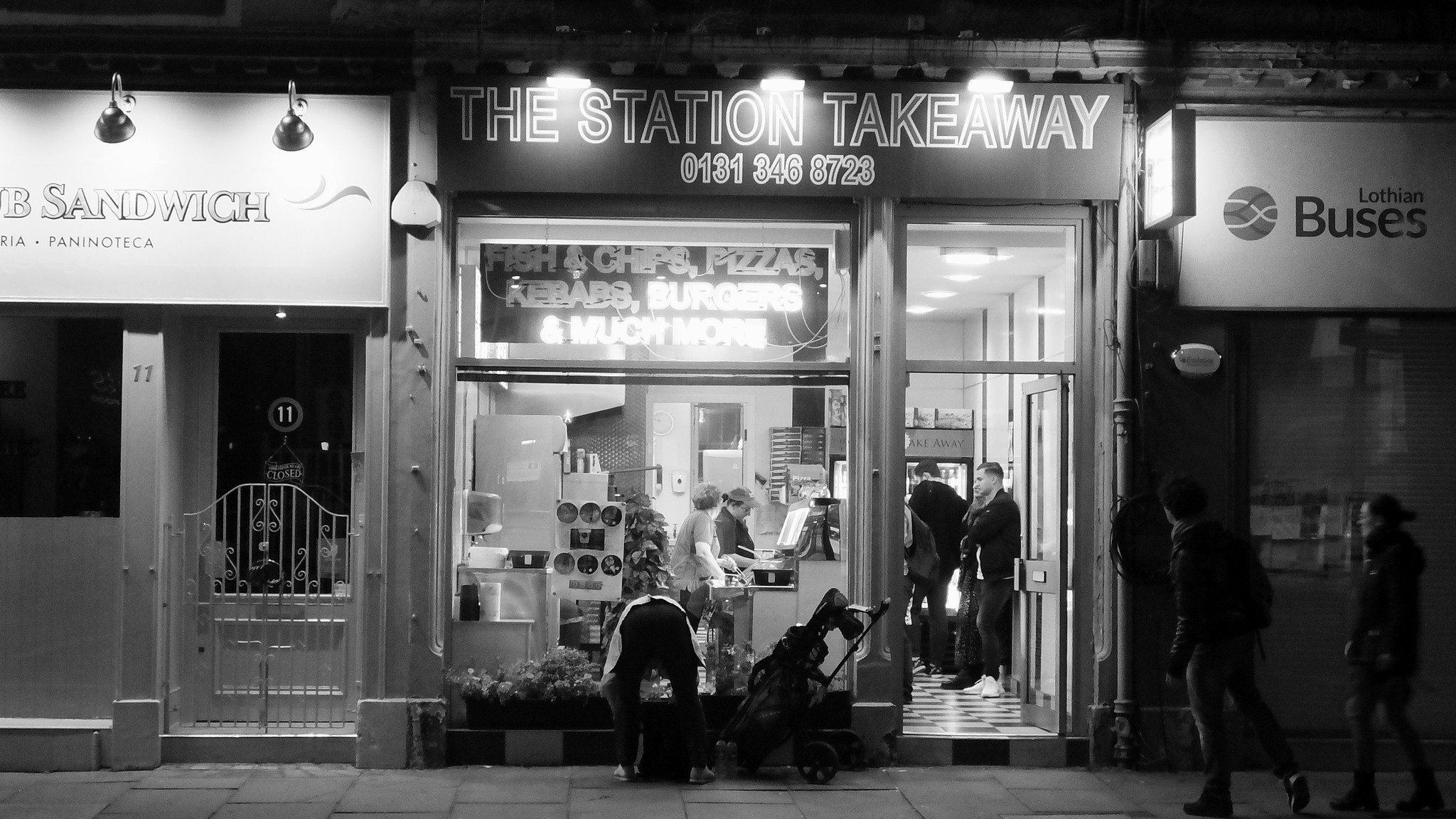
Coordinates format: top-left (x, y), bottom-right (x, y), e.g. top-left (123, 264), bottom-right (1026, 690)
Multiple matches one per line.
top-left (716, 590), bottom-right (890, 786)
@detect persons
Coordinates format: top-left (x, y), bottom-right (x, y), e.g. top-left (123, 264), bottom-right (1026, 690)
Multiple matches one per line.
top-left (1327, 497), bottom-right (1447, 813)
top-left (902, 462), bottom-right (1021, 703)
top-left (670, 481), bottom-right (736, 635)
top-left (601, 594), bottom-right (716, 785)
top-left (714, 486), bottom-right (760, 574)
top-left (1159, 478), bottom-right (1310, 817)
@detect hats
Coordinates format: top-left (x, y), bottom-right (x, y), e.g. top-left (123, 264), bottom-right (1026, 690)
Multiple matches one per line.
top-left (729, 486), bottom-right (762, 508)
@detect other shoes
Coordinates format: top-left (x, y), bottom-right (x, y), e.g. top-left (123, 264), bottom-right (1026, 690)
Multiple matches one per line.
top-left (940, 673), bottom-right (980, 690)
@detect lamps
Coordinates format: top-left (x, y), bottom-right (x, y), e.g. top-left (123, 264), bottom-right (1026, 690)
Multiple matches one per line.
top-left (271, 79), bottom-right (314, 151)
top-left (93, 71), bottom-right (138, 144)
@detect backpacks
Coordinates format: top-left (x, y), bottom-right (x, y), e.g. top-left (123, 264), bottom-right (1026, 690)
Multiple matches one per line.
top-left (1172, 535), bottom-right (1274, 661)
top-left (903, 501), bottom-right (942, 587)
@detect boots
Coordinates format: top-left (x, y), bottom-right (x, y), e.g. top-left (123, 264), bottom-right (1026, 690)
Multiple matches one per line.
top-left (1330, 767), bottom-right (1380, 812)
top-left (1396, 765), bottom-right (1445, 810)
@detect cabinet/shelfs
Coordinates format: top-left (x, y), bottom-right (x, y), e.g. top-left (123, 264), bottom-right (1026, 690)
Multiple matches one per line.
top-left (450, 620), bottom-right (535, 723)
top-left (455, 565), bottom-right (553, 681)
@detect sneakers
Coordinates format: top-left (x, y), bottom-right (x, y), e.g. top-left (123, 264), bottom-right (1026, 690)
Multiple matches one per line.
top-left (614, 763), bottom-right (636, 781)
top-left (1283, 770), bottom-right (1310, 813)
top-left (930, 665), bottom-right (943, 678)
top-left (963, 674), bottom-right (1006, 698)
top-left (1183, 787), bottom-right (1234, 818)
top-left (690, 765), bottom-right (715, 783)
top-left (912, 658), bottom-right (926, 675)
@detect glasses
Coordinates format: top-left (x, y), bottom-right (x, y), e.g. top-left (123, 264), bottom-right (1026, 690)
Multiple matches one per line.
top-left (737, 503), bottom-right (754, 514)
top-left (720, 495), bottom-right (724, 504)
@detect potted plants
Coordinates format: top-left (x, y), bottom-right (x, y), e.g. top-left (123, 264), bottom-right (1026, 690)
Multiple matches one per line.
top-left (447, 648), bottom-right (850, 729)
